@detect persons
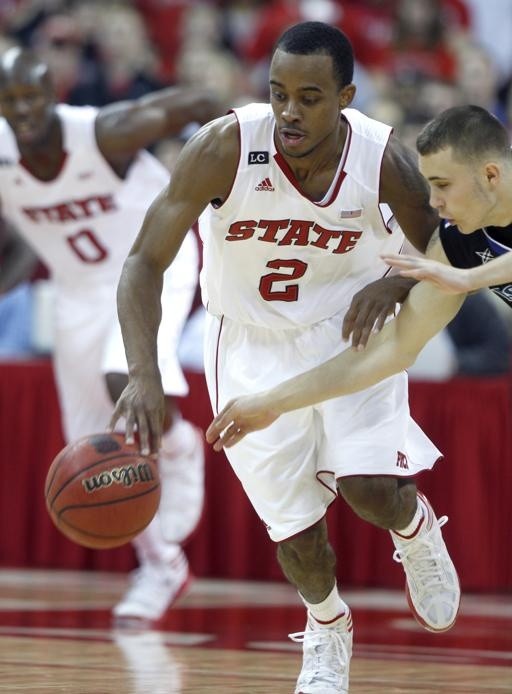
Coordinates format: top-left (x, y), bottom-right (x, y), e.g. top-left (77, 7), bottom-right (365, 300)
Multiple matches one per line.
top-left (0, 1), bottom-right (510, 374)
top-left (0, 46), bottom-right (234, 619)
top-left (109, 24), bottom-right (512, 692)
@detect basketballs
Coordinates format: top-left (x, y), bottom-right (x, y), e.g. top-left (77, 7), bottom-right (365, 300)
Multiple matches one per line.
top-left (45, 434), bottom-right (162, 549)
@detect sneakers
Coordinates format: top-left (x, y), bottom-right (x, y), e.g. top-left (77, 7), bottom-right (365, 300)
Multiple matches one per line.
top-left (293, 597), bottom-right (354, 693)
top-left (388, 489), bottom-right (462, 635)
top-left (110, 546), bottom-right (191, 624)
